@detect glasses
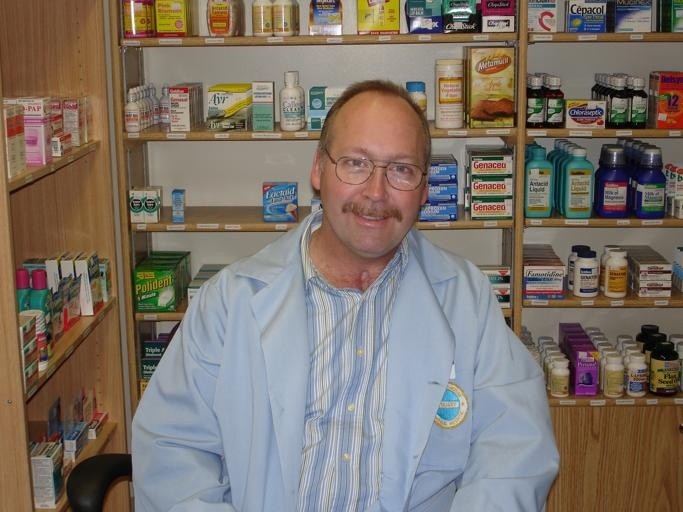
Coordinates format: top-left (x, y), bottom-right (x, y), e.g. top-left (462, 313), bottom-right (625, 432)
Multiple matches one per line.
top-left (320, 142), bottom-right (430, 193)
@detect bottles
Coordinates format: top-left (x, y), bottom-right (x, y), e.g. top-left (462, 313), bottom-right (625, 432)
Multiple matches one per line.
top-left (124, 81), bottom-right (171, 132)
top-left (278, 71), bottom-right (307, 131)
top-left (433, 59), bottom-right (464, 129)
top-left (17, 263), bottom-right (56, 376)
top-left (526, 139), bottom-right (683, 219)
top-left (568, 242), bottom-right (631, 299)
top-left (406, 82), bottom-right (428, 120)
top-left (526, 67), bottom-right (650, 127)
top-left (518, 320), bottom-right (682, 398)
top-left (123, 1), bottom-right (297, 39)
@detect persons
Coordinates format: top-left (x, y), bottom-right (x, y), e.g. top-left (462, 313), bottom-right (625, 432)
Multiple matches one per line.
top-left (130, 80), bottom-right (560, 512)
